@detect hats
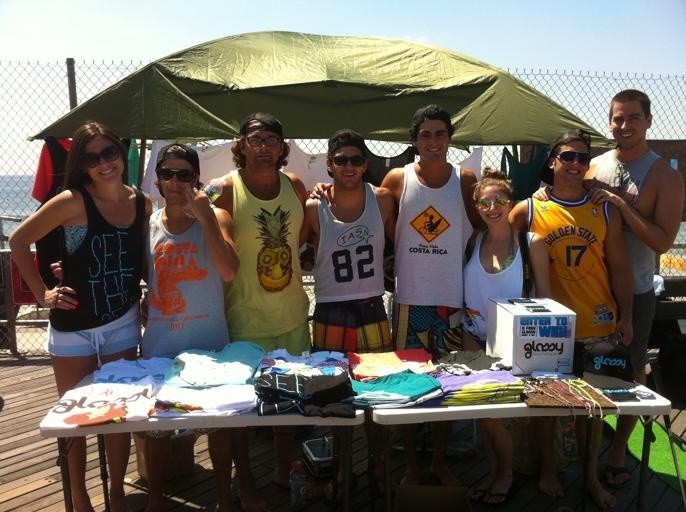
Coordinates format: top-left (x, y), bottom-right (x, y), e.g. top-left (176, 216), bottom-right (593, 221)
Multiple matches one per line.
top-left (240, 113), bottom-right (283, 135)
top-left (540, 129), bottom-right (591, 185)
top-left (156, 144), bottom-right (200, 172)
top-left (328, 129), bottom-right (363, 144)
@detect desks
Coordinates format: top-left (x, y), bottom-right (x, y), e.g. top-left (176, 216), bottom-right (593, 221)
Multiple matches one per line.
top-left (40, 354), bottom-right (672, 511)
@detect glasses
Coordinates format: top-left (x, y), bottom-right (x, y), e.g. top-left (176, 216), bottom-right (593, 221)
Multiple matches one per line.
top-left (557, 151), bottom-right (591, 166)
top-left (80, 145), bottom-right (119, 168)
top-left (478, 194), bottom-right (508, 211)
top-left (333, 156), bottom-right (364, 166)
top-left (156, 169), bottom-right (194, 182)
top-left (246, 136), bottom-right (279, 147)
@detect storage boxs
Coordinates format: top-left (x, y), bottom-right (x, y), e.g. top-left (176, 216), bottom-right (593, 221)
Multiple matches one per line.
top-left (487, 295), bottom-right (577, 375)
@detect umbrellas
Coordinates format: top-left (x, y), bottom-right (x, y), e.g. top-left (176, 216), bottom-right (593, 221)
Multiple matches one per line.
top-left (22, 29), bottom-right (628, 158)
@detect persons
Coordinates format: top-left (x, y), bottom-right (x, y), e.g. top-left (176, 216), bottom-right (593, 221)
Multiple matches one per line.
top-left (297, 127), bottom-right (399, 510)
top-left (307, 100), bottom-right (486, 510)
top-left (505, 127), bottom-right (637, 511)
top-left (137, 113), bottom-right (313, 512)
top-left (460, 161), bottom-right (557, 508)
top-left (140, 142), bottom-right (242, 512)
top-left (7, 123), bottom-right (153, 511)
top-left (530, 90), bottom-right (686, 490)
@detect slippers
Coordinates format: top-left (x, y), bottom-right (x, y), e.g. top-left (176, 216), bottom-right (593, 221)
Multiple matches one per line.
top-left (606, 465), bottom-right (631, 486)
top-left (468, 477), bottom-right (514, 505)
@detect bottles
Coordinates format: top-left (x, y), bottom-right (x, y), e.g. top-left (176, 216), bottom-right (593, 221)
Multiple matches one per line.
top-left (182, 175), bottom-right (225, 220)
top-left (288, 460), bottom-right (306, 511)
